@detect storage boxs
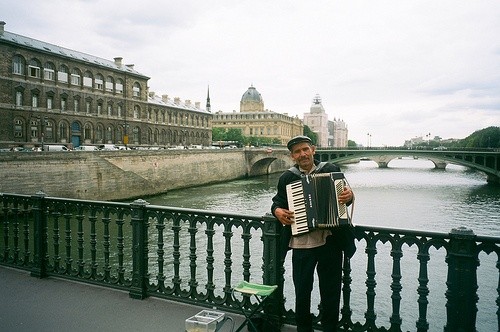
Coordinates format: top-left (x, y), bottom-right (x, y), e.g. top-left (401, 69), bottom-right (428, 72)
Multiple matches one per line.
top-left (185, 310), bottom-right (226, 332)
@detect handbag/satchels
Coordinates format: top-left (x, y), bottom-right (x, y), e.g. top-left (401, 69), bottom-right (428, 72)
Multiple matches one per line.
top-left (247, 313), bottom-right (280, 332)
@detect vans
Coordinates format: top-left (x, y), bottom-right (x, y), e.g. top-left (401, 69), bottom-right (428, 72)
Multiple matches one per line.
top-left (192, 145), bottom-right (202, 149)
top-left (47, 146), bottom-right (72, 152)
top-left (115, 146), bottom-right (125, 150)
top-left (79, 145), bottom-right (98, 151)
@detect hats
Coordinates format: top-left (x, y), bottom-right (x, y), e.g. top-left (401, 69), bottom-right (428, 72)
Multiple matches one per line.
top-left (286, 136), bottom-right (313, 152)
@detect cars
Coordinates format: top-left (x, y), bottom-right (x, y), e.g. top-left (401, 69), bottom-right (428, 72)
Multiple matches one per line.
top-left (0, 146), bottom-right (42, 152)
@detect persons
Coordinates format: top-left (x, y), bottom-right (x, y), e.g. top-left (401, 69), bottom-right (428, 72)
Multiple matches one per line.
top-left (271, 135), bottom-right (354, 332)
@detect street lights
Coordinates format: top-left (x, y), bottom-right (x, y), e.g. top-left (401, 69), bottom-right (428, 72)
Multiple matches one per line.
top-left (41, 133), bottom-right (44, 152)
top-left (370, 134), bottom-right (371, 150)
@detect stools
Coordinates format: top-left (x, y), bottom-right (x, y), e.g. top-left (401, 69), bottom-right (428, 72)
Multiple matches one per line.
top-left (231, 281), bottom-right (278, 332)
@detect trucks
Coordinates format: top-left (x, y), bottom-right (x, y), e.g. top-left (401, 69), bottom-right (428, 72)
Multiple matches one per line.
top-left (99, 144), bottom-right (115, 150)
